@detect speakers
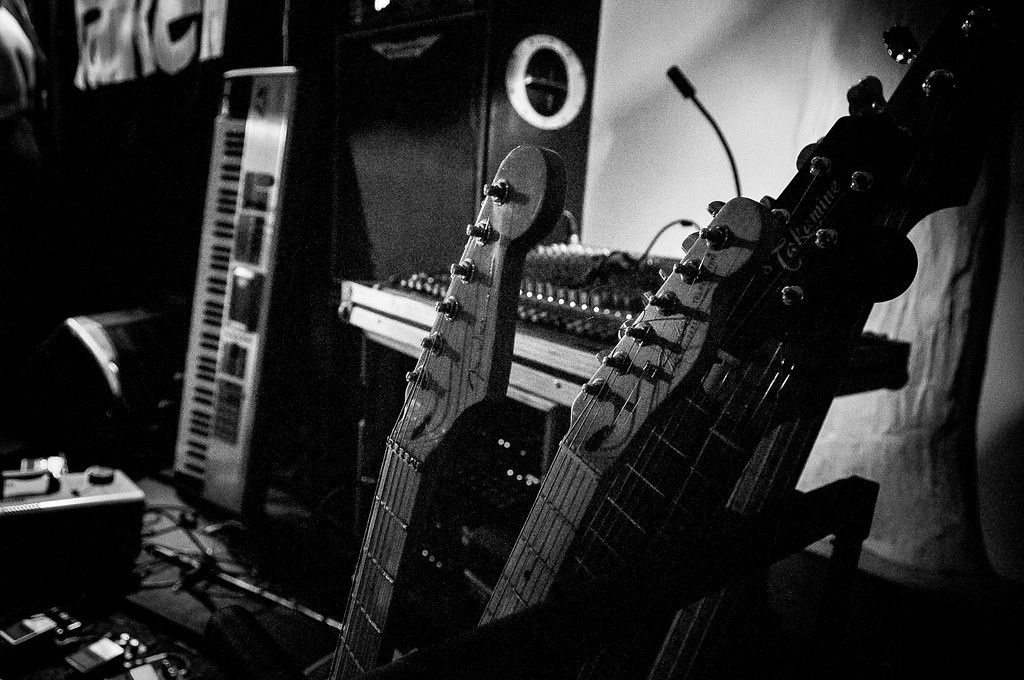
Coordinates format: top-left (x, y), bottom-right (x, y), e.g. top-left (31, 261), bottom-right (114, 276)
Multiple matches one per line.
top-left (323, 9), bottom-right (599, 287)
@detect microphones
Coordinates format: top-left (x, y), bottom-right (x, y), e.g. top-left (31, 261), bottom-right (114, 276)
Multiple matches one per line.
top-left (668, 65), bottom-right (741, 198)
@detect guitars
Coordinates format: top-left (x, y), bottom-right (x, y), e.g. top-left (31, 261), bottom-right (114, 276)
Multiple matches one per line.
top-left (327, 141), bottom-right (567, 680)
top-left (475, 7), bottom-right (1000, 680)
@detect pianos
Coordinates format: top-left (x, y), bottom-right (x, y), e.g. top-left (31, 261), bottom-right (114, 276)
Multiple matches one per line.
top-left (171, 66), bottom-right (317, 513)
